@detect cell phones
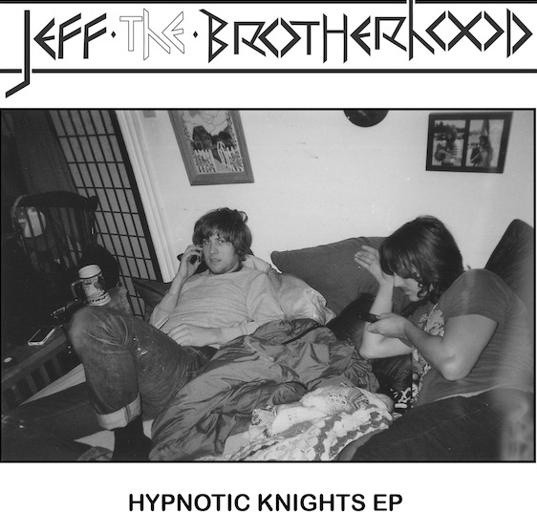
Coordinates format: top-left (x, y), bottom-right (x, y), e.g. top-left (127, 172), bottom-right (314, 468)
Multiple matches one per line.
top-left (27, 328), bottom-right (55, 346)
top-left (190, 246), bottom-right (202, 264)
top-left (357, 312), bottom-right (380, 323)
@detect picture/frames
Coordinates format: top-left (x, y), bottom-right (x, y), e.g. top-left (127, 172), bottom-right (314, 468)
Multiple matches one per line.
top-left (167, 109), bottom-right (253, 187)
top-left (423, 112), bottom-right (513, 174)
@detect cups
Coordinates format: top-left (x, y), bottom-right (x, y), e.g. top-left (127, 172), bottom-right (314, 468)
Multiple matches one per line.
top-left (71, 264), bottom-right (112, 307)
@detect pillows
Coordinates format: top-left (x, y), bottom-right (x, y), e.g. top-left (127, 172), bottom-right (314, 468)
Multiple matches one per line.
top-left (266, 236), bottom-right (415, 321)
top-left (133, 275), bottom-right (170, 313)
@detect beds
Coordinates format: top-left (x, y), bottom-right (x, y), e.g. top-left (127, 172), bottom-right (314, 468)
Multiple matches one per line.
top-left (1, 330), bottom-right (536, 463)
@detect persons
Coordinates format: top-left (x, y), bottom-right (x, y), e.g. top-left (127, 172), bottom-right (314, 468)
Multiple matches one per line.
top-left (335, 218), bottom-right (533, 460)
top-left (68, 208), bottom-right (284, 461)
